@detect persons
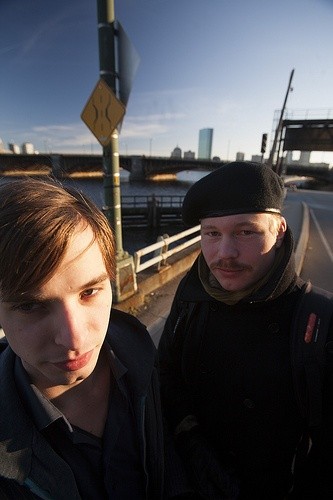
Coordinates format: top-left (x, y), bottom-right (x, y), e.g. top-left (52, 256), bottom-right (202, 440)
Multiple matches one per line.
top-left (0, 175), bottom-right (162, 500)
top-left (158, 161), bottom-right (333, 500)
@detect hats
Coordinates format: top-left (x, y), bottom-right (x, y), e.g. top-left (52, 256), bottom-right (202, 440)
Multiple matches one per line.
top-left (181, 161), bottom-right (285, 226)
